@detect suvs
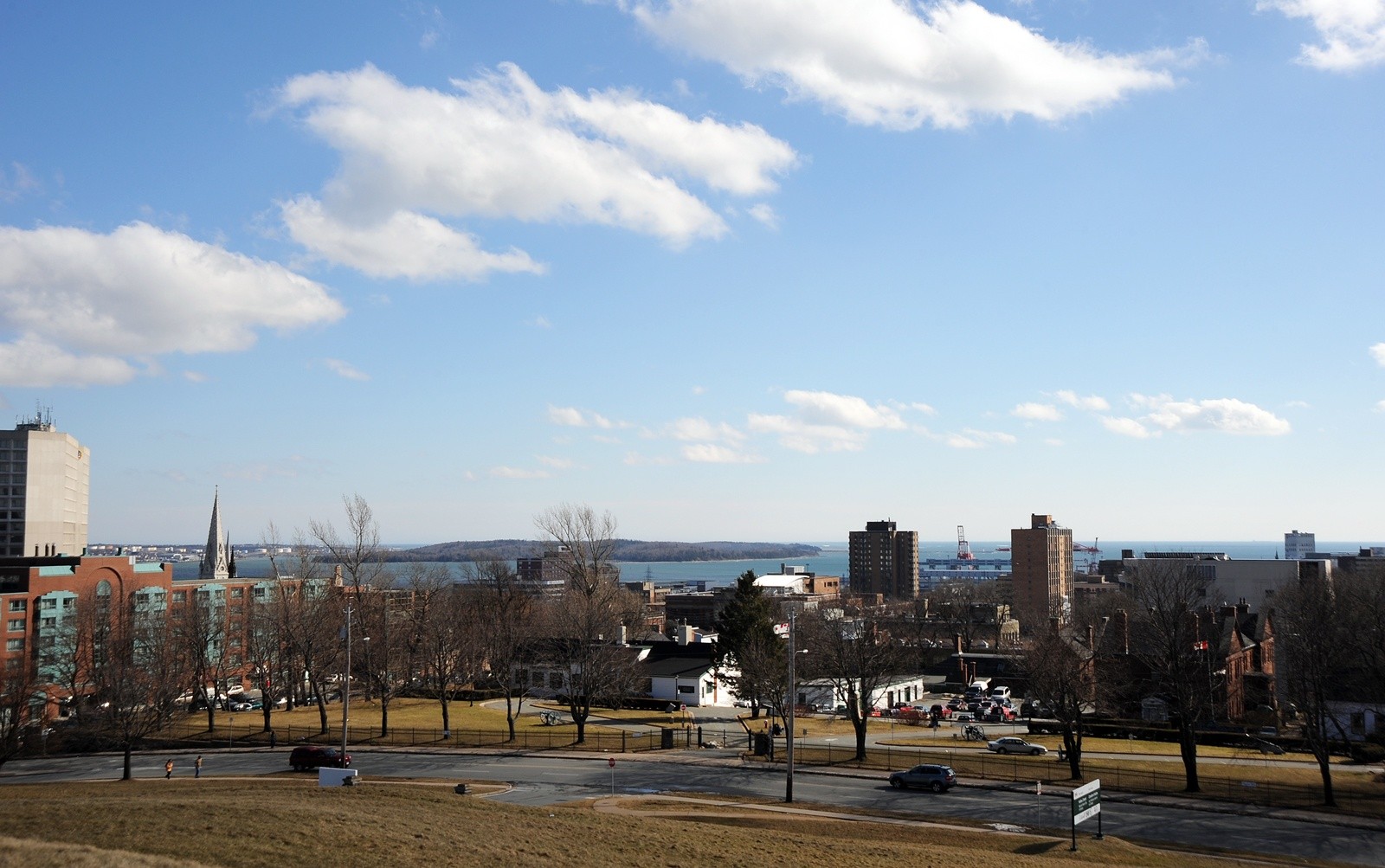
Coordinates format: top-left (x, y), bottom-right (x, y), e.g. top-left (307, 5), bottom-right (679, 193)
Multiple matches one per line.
top-left (289, 746), bottom-right (352, 773)
top-left (889, 763), bottom-right (958, 792)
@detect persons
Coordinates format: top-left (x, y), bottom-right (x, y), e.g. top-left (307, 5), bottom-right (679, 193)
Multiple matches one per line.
top-left (165, 758), bottom-right (173, 779)
top-left (270, 730), bottom-right (276, 749)
top-left (195, 755), bottom-right (203, 778)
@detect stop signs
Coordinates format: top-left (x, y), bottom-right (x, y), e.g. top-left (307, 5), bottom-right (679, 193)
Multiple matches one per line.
top-left (608, 758), bottom-right (616, 767)
top-left (680, 704), bottom-right (687, 712)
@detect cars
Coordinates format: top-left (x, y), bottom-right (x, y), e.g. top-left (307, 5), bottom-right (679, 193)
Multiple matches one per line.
top-left (173, 685), bottom-right (263, 711)
top-left (987, 736), bottom-right (1047, 756)
top-left (1020, 697), bottom-right (1056, 719)
top-left (325, 673), bottom-right (353, 684)
top-left (885, 702), bottom-right (952, 720)
top-left (733, 696), bottom-right (759, 709)
top-left (807, 704), bottom-right (846, 715)
top-left (277, 695), bottom-right (295, 704)
top-left (946, 681), bottom-right (1018, 722)
top-left (307, 694), bottom-right (319, 705)
top-left (860, 706), bottom-right (881, 717)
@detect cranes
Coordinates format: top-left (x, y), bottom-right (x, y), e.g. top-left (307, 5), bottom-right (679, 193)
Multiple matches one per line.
top-left (957, 525), bottom-right (973, 560)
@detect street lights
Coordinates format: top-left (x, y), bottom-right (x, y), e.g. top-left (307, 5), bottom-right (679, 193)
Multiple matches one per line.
top-left (786, 648), bottom-right (810, 803)
top-left (341, 636), bottom-right (371, 768)
